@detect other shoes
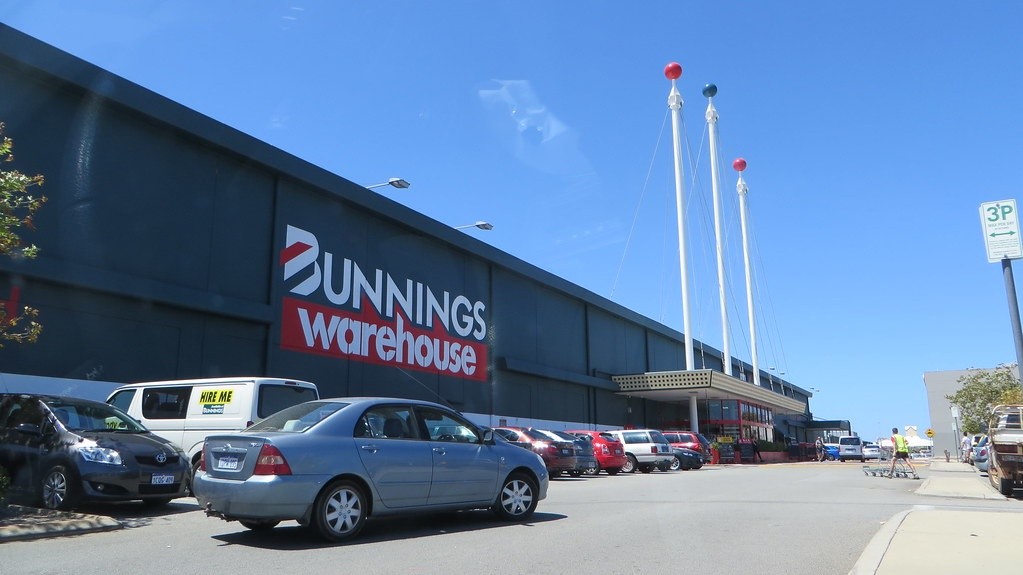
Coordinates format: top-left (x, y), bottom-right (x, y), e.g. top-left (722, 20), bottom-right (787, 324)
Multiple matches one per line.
top-left (914, 475), bottom-right (919, 479)
top-left (888, 474), bottom-right (892, 479)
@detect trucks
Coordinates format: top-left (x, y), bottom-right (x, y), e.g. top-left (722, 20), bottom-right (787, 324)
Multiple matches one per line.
top-left (985, 404), bottom-right (1023, 496)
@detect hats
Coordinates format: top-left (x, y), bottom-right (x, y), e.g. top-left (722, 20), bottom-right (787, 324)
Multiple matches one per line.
top-left (892, 428), bottom-right (898, 432)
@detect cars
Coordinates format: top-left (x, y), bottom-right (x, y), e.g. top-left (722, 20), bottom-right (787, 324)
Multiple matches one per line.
top-left (667, 447), bottom-right (705, 471)
top-left (864, 443), bottom-right (886, 461)
top-left (1, 392), bottom-right (192, 513)
top-left (908, 449), bottom-right (931, 459)
top-left (969, 435), bottom-right (985, 465)
top-left (562, 430), bottom-right (627, 476)
top-left (429, 425), bottom-right (477, 443)
top-left (824, 445), bottom-right (839, 461)
top-left (191, 397), bottom-right (549, 545)
top-left (486, 425), bottom-right (578, 480)
top-left (534, 429), bottom-right (597, 478)
top-left (974, 436), bottom-right (988, 473)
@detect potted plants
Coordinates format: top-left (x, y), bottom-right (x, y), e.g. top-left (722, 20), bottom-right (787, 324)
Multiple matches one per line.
top-left (709, 442), bottom-right (720, 463)
top-left (732, 443), bottom-right (741, 462)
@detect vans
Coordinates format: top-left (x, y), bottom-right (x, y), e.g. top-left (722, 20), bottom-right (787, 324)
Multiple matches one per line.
top-left (104, 377), bottom-right (320, 496)
top-left (838, 436), bottom-right (865, 462)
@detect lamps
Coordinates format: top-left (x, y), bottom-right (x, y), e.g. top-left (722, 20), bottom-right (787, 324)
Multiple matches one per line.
top-left (763, 367), bottom-right (786, 375)
top-left (366, 177), bottom-right (410, 188)
top-left (455, 221), bottom-right (494, 231)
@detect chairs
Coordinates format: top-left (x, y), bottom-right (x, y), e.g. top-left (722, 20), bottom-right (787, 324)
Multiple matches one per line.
top-left (53, 409), bottom-right (69, 427)
top-left (382, 418), bottom-right (403, 438)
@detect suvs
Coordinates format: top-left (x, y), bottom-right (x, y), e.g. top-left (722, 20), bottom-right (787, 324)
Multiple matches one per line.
top-left (603, 429), bottom-right (675, 474)
top-left (662, 431), bottom-right (710, 462)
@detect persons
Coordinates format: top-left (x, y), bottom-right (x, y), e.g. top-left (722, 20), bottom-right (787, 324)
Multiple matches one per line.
top-left (816, 437), bottom-right (824, 463)
top-left (887, 428), bottom-right (919, 479)
top-left (961, 432), bottom-right (971, 463)
top-left (752, 436), bottom-right (764, 462)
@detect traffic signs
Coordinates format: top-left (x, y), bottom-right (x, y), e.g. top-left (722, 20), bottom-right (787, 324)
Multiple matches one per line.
top-left (981, 199), bottom-right (1023, 260)
